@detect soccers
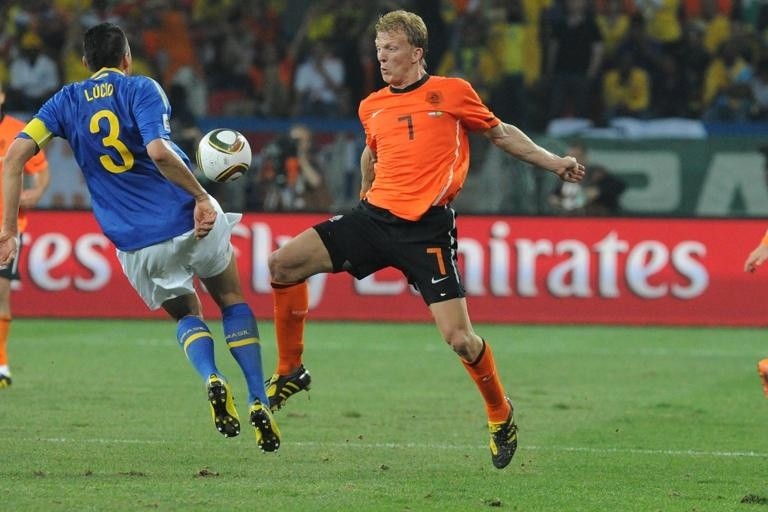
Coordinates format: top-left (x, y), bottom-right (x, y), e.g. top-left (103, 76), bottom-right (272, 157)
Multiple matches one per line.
top-left (196, 128), bottom-right (252, 183)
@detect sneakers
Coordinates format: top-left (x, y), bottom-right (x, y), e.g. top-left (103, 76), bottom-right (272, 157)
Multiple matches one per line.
top-left (249, 404), bottom-right (282, 454)
top-left (206, 376), bottom-right (240, 438)
top-left (487, 396), bottom-right (517, 469)
top-left (264, 363), bottom-right (313, 413)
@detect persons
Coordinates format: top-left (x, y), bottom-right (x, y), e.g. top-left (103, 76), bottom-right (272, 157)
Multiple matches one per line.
top-left (744, 224), bottom-right (768, 398)
top-left (258, 9), bottom-right (585, 469)
top-left (0, 20), bottom-right (283, 452)
top-left (1, 90), bottom-right (50, 389)
top-left (0, 0), bottom-right (768, 128)
top-left (546, 141), bottom-right (623, 215)
top-left (261, 123), bottom-right (321, 213)
